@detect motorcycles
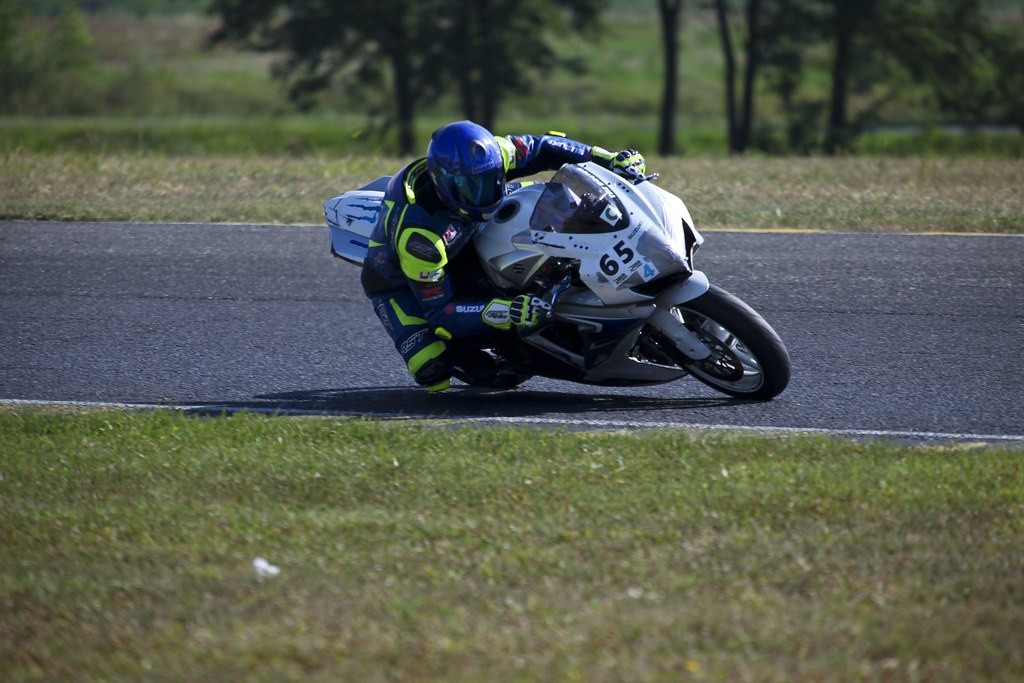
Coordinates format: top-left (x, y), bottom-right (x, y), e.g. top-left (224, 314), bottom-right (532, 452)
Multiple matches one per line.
top-left (322, 160), bottom-right (793, 401)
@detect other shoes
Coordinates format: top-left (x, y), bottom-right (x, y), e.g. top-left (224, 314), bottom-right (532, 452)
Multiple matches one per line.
top-left (444, 344), bottom-right (496, 381)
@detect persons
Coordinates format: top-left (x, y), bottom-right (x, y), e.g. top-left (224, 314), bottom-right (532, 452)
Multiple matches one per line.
top-left (359, 117), bottom-right (644, 395)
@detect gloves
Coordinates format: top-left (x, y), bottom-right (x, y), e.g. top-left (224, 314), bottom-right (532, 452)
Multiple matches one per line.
top-left (483, 295), bottom-right (551, 333)
top-left (591, 146), bottom-right (647, 180)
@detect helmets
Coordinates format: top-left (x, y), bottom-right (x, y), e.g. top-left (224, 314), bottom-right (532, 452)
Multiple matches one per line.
top-left (426, 119), bottom-right (506, 223)
